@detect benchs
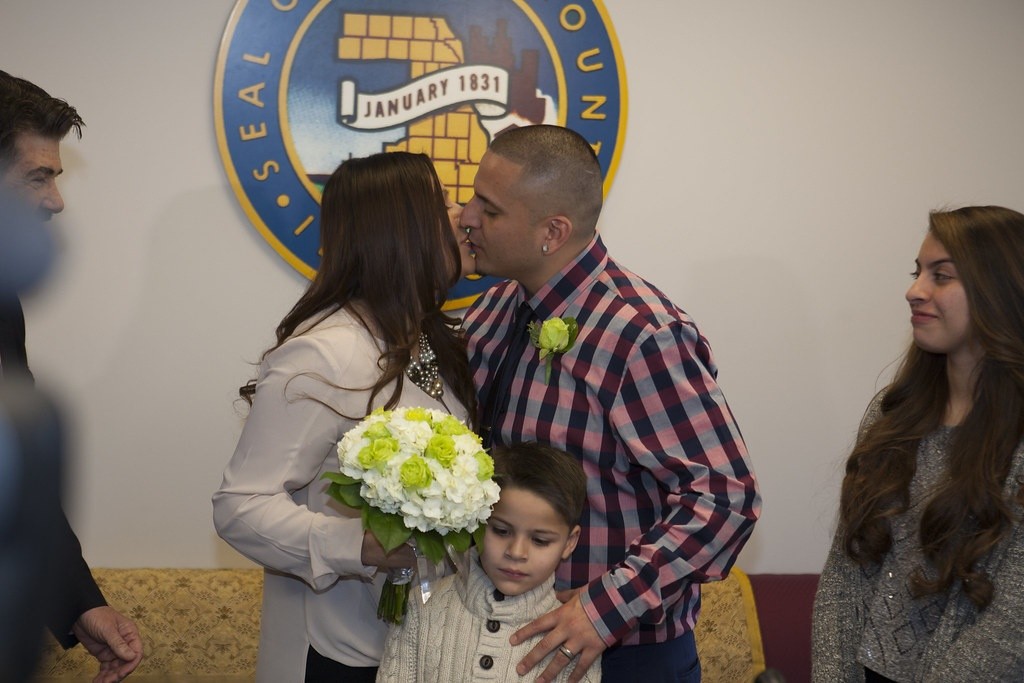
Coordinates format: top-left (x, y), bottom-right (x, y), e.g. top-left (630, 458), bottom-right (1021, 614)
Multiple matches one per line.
top-left (29, 567), bottom-right (780, 683)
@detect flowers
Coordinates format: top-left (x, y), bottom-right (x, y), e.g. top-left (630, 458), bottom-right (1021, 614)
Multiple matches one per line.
top-left (324, 406), bottom-right (501, 627)
top-left (527, 317), bottom-right (578, 385)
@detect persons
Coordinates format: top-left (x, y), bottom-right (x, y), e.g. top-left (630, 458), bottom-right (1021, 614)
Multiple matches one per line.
top-left (461, 126), bottom-right (764, 683)
top-left (211, 151), bottom-right (477, 683)
top-left (0, 69), bottom-right (146, 683)
top-left (373, 440), bottom-right (605, 683)
top-left (807, 204), bottom-right (1024, 683)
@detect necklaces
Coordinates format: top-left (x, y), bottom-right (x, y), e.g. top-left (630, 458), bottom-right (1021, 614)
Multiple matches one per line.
top-left (403, 332), bottom-right (444, 397)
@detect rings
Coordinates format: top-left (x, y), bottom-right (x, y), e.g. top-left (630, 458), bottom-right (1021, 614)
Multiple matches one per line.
top-left (558, 644), bottom-right (575, 661)
